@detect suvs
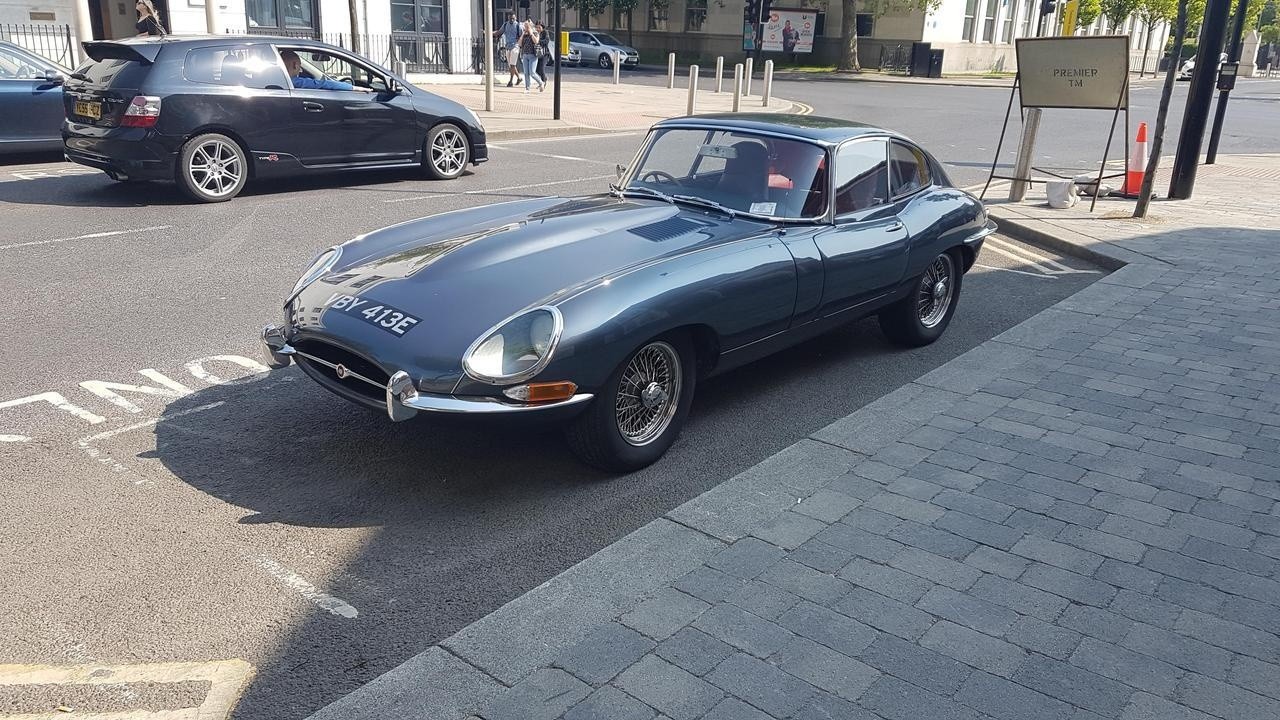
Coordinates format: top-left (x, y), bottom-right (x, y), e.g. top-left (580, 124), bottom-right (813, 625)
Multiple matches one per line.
top-left (567, 30), bottom-right (641, 70)
top-left (496, 30), bottom-right (583, 67)
top-left (60, 34), bottom-right (491, 205)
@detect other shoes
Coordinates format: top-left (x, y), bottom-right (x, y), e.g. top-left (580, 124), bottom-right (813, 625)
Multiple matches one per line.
top-left (516, 78), bottom-right (523, 85)
top-left (507, 82), bottom-right (513, 87)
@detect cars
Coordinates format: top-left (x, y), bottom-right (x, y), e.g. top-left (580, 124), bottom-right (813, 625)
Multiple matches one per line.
top-left (1180, 53), bottom-right (1229, 81)
top-left (0, 38), bottom-right (75, 155)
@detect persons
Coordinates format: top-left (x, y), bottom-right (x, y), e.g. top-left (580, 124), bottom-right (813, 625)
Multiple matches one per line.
top-left (493, 11), bottom-right (523, 88)
top-left (134, 0), bottom-right (168, 35)
top-left (280, 50), bottom-right (374, 94)
top-left (782, 20), bottom-right (800, 51)
top-left (394, 11), bottom-right (438, 64)
top-left (517, 14), bottom-right (546, 94)
top-left (534, 19), bottom-right (550, 89)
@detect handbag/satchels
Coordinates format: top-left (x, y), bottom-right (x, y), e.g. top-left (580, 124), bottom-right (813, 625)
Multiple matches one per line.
top-left (534, 47), bottom-right (543, 57)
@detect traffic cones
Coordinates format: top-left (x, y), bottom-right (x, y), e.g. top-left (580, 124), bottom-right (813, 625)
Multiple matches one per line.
top-left (1106, 122), bottom-right (1159, 200)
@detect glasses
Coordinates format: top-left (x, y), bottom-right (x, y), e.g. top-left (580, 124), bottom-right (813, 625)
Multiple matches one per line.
top-left (138, 2), bottom-right (144, 5)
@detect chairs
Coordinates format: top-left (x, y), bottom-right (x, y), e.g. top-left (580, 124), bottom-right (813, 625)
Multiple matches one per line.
top-left (719, 141), bottom-right (771, 202)
top-left (812, 148), bottom-right (881, 217)
top-left (219, 56), bottom-right (247, 85)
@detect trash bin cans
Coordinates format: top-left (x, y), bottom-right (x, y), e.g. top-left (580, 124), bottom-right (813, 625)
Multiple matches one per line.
top-left (912, 42), bottom-right (931, 77)
top-left (928, 49), bottom-right (944, 77)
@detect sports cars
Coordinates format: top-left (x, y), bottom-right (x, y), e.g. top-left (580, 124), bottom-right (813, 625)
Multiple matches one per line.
top-left (257, 110), bottom-right (999, 474)
